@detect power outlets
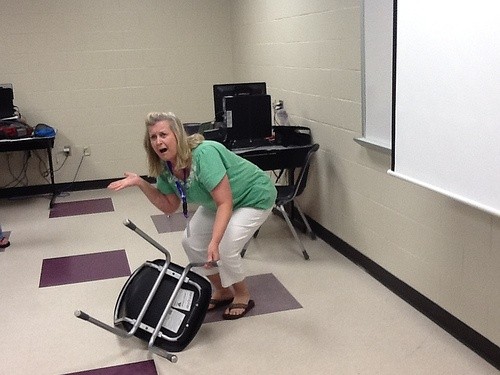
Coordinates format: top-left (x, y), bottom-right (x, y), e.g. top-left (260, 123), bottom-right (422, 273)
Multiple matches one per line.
top-left (83, 145), bottom-right (90, 156)
top-left (64, 145), bottom-right (72, 156)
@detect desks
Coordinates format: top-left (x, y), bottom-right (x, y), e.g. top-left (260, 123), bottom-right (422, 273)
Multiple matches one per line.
top-left (235, 144), bottom-right (316, 240)
top-left (0, 135), bottom-right (61, 208)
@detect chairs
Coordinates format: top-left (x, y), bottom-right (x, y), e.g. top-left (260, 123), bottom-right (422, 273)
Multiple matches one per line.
top-left (74, 218), bottom-right (233, 363)
top-left (240, 143), bottom-right (320, 261)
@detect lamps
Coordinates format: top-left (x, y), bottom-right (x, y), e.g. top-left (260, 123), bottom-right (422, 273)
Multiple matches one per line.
top-left (272, 99), bottom-right (288, 125)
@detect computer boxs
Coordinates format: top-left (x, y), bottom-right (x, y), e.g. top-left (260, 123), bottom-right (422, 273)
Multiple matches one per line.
top-left (0, 87), bottom-right (16, 118)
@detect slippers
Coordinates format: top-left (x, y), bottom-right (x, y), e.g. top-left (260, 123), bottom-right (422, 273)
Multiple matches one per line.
top-left (223, 299), bottom-right (255, 320)
top-left (207, 297), bottom-right (235, 312)
top-left (0, 237), bottom-right (10, 248)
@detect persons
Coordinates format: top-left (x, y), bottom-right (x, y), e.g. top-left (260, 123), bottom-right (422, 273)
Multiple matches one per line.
top-left (106, 111), bottom-right (277, 321)
top-left (0, 226), bottom-right (10, 249)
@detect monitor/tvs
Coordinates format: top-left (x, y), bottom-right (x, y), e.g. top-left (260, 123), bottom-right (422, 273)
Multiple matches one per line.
top-left (225, 95), bottom-right (274, 148)
top-left (214, 81), bottom-right (267, 142)
